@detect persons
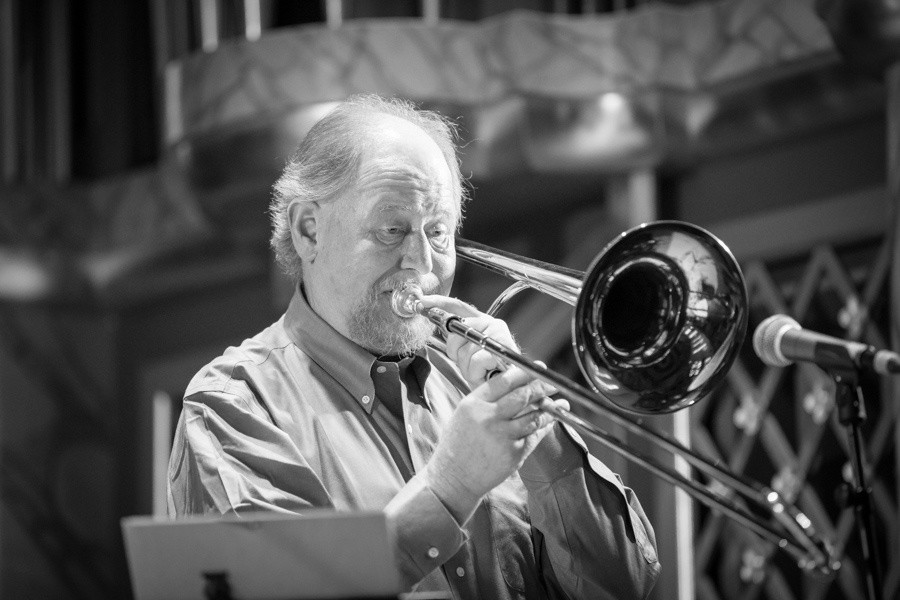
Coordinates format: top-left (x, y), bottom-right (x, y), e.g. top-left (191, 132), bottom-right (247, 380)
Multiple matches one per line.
top-left (166, 92), bottom-right (662, 600)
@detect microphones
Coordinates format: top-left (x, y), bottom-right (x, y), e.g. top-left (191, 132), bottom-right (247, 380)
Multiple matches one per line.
top-left (753, 313), bottom-right (900, 375)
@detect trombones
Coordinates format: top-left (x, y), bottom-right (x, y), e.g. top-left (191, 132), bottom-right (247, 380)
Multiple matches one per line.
top-left (390, 221), bottom-right (841, 579)
